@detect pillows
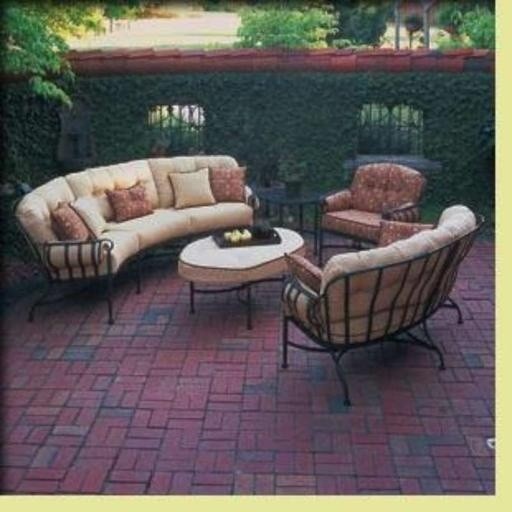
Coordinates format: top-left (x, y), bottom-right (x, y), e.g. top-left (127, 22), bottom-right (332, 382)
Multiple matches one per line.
top-left (55, 166), bottom-right (247, 242)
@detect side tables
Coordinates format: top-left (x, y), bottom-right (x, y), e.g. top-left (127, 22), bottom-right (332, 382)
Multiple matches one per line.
top-left (258, 187), bottom-right (324, 256)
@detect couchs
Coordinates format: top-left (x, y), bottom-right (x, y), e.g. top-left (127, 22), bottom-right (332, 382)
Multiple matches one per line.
top-left (16, 154), bottom-right (262, 325)
top-left (282, 203), bottom-right (484, 406)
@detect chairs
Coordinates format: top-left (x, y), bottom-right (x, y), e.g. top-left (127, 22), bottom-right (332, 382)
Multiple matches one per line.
top-left (318, 162), bottom-right (425, 266)
